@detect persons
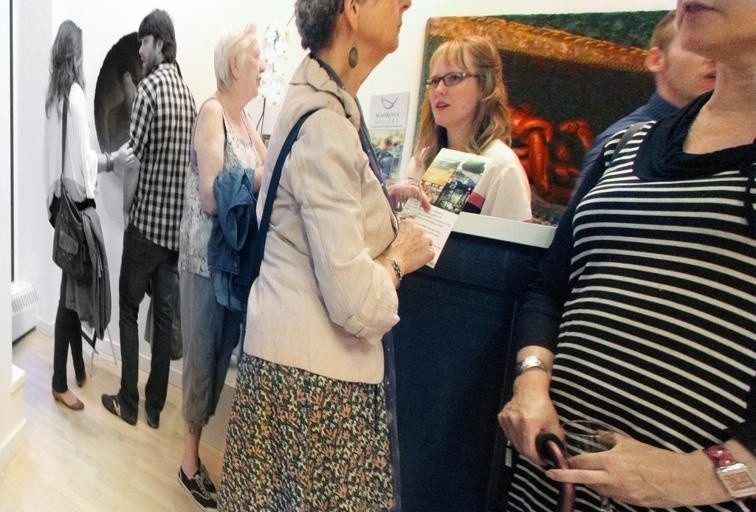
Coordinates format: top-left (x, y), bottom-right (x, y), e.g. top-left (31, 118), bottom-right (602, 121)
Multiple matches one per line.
top-left (101, 8), bottom-right (197, 429)
top-left (491, 0), bottom-right (756, 511)
top-left (170, 18), bottom-right (270, 511)
top-left (216, 1), bottom-right (436, 512)
top-left (43, 17), bottom-right (136, 412)
top-left (582, 7), bottom-right (719, 181)
top-left (394, 32), bottom-right (533, 224)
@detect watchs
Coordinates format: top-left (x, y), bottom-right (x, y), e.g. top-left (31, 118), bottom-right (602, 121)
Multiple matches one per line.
top-left (699, 444), bottom-right (756, 501)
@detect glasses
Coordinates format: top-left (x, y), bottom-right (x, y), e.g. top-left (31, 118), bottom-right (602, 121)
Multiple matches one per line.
top-left (425, 71), bottom-right (482, 91)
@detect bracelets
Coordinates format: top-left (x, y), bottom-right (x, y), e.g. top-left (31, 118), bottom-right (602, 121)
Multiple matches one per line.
top-left (103, 151), bottom-right (110, 173)
top-left (511, 353), bottom-right (550, 378)
top-left (379, 253), bottom-right (402, 290)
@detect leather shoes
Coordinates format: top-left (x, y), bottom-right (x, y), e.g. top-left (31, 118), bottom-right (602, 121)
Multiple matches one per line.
top-left (52, 387), bottom-right (84, 409)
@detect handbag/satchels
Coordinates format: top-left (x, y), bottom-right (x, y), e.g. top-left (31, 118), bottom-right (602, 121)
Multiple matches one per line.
top-left (53, 183), bottom-right (94, 288)
top-left (144, 272), bottom-right (183, 359)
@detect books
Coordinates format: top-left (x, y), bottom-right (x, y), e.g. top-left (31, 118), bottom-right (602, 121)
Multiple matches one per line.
top-left (396, 147), bottom-right (493, 272)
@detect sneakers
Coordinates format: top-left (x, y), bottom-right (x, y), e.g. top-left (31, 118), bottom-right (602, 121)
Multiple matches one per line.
top-left (102, 394), bottom-right (137, 425)
top-left (145, 401), bottom-right (159, 428)
top-left (199, 462), bottom-right (217, 500)
top-left (178, 465), bottom-right (217, 512)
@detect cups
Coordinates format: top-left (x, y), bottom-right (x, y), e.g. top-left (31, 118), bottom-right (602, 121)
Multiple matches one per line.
top-left (561, 419), bottom-right (614, 459)
